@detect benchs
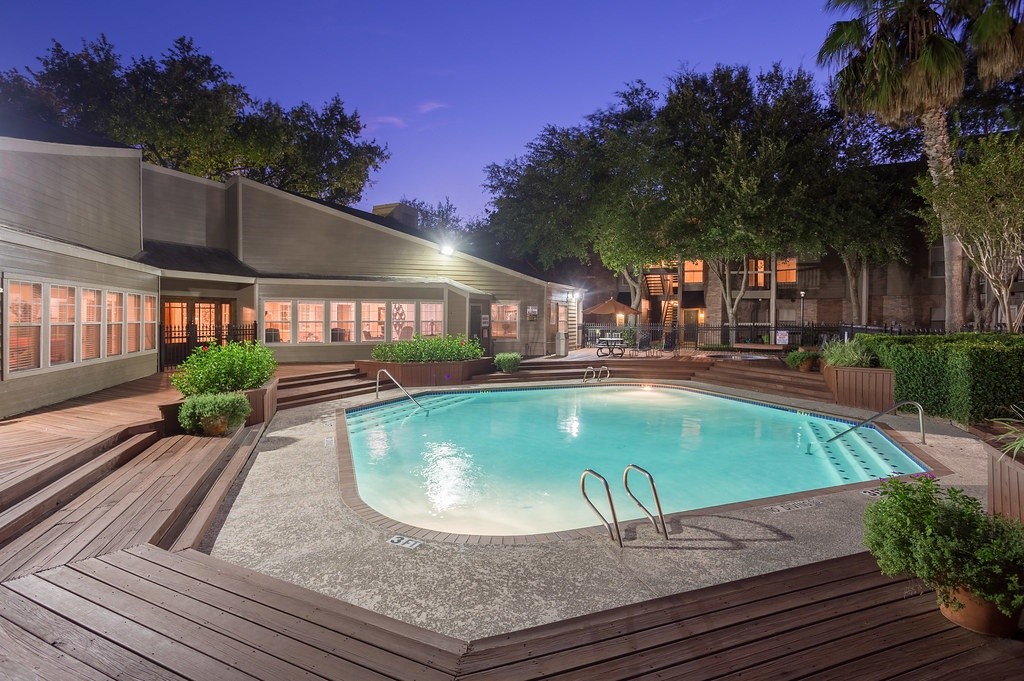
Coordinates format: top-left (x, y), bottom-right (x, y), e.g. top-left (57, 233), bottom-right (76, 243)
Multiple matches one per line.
top-left (595, 343), bottom-right (628, 357)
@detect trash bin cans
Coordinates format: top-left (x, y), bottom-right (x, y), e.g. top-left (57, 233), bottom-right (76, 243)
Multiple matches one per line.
top-left (555, 332), bottom-right (570, 357)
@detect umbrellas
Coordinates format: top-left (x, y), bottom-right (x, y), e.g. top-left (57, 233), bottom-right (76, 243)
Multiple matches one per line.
top-left (582, 296), bottom-right (643, 346)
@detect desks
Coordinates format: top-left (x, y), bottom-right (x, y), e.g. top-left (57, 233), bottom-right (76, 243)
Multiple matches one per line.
top-left (599, 337), bottom-right (623, 356)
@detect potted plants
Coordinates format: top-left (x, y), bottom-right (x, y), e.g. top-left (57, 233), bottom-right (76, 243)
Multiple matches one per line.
top-left (157, 335), bottom-right (280, 436)
top-left (354, 333), bottom-right (492, 386)
top-left (785, 350), bottom-right (821, 372)
top-left (177, 391), bottom-right (255, 436)
top-left (494, 351), bottom-right (523, 372)
top-left (861, 470), bottom-right (1024, 634)
